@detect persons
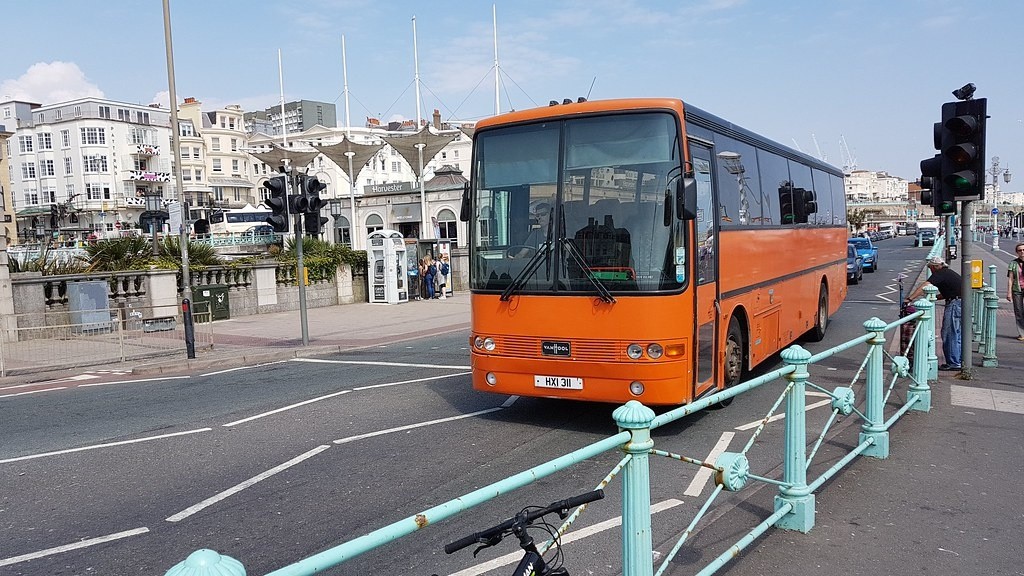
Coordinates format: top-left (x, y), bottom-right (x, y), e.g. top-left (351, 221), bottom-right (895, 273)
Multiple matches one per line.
top-left (1006, 244), bottom-right (1024, 341)
top-left (419, 253), bottom-right (447, 300)
top-left (515, 203), bottom-right (571, 259)
top-left (979, 225), bottom-right (1009, 238)
top-left (906, 257), bottom-right (962, 371)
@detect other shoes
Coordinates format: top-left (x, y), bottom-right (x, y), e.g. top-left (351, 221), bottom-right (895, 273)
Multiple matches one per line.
top-left (438, 296), bottom-right (446, 300)
top-left (1018, 334), bottom-right (1024, 341)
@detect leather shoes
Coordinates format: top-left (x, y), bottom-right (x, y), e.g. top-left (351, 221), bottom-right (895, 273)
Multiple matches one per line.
top-left (939, 363), bottom-right (961, 371)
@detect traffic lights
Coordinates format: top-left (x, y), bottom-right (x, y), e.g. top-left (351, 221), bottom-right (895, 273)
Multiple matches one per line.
top-left (920, 156), bottom-right (958, 216)
top-left (301, 174), bottom-right (322, 234)
top-left (286, 192), bottom-right (308, 215)
top-left (319, 183), bottom-right (330, 227)
top-left (942, 96), bottom-right (986, 198)
top-left (262, 175), bottom-right (289, 231)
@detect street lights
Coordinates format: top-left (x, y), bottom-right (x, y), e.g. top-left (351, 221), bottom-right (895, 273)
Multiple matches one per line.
top-left (986, 155), bottom-right (1012, 251)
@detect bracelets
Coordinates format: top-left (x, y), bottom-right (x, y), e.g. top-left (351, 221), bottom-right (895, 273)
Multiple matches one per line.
top-left (907, 297), bottom-right (912, 302)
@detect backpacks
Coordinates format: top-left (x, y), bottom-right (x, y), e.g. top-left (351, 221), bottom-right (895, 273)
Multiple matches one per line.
top-left (430, 265), bottom-right (437, 275)
top-left (438, 260), bottom-right (449, 275)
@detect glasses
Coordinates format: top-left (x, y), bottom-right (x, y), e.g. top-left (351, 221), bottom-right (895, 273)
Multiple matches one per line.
top-left (1017, 249), bottom-right (1024, 251)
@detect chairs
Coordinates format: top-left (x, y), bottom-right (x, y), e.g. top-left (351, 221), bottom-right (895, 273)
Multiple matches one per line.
top-left (558, 198), bottom-right (666, 242)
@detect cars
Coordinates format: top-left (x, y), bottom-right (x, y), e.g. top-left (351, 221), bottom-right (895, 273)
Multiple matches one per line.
top-left (868, 231), bottom-right (892, 242)
top-left (848, 237), bottom-right (878, 273)
top-left (846, 244), bottom-right (863, 284)
top-left (913, 230), bottom-right (935, 247)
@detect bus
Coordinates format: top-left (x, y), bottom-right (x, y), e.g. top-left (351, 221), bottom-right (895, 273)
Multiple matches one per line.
top-left (879, 224), bottom-right (916, 237)
top-left (207, 209), bottom-right (274, 233)
top-left (459, 99), bottom-right (848, 412)
